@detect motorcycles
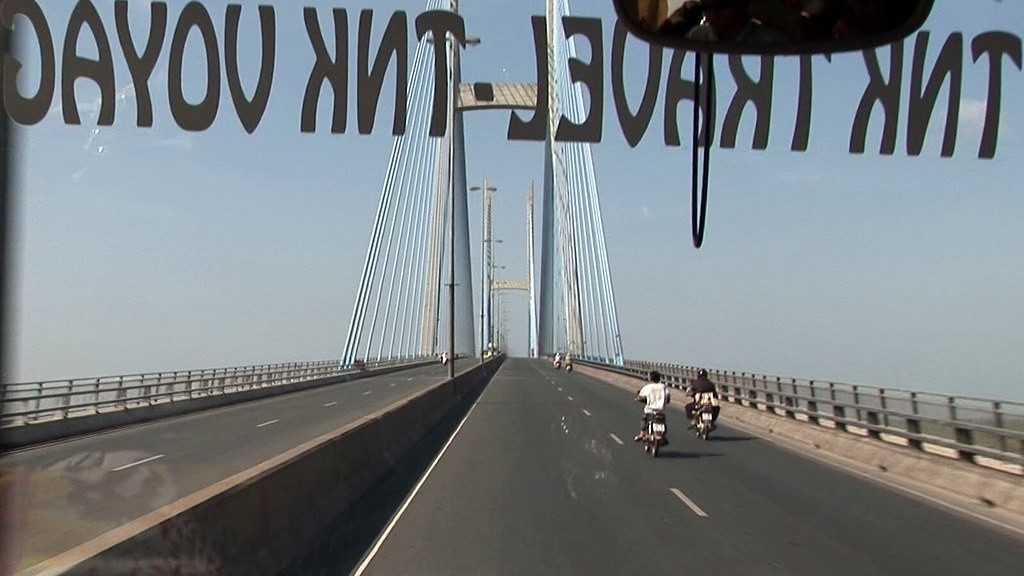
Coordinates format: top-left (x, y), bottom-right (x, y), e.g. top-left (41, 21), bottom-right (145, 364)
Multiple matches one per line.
top-left (633, 380), bottom-right (669, 458)
top-left (685, 377), bottom-right (719, 441)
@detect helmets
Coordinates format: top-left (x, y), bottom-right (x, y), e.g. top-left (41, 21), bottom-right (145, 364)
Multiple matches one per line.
top-left (697, 369), bottom-right (707, 378)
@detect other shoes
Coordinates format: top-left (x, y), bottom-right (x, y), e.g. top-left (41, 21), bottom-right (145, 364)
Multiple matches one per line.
top-left (633, 436), bottom-right (644, 441)
top-left (662, 436), bottom-right (669, 445)
top-left (708, 423), bottom-right (714, 427)
top-left (687, 424), bottom-right (697, 430)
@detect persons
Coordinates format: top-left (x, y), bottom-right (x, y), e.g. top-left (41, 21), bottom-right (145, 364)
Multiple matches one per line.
top-left (633, 370), bottom-right (670, 444)
top-left (564, 352), bottom-right (573, 371)
top-left (684, 368), bottom-right (721, 431)
top-left (553, 353), bottom-right (561, 368)
top-left (440, 352), bottom-right (448, 364)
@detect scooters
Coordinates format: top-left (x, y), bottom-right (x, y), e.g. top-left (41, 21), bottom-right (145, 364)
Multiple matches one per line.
top-left (555, 360), bottom-right (561, 369)
top-left (566, 364), bottom-right (573, 374)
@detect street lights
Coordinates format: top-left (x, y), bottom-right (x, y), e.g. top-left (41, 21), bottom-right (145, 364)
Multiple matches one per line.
top-left (470, 182), bottom-right (508, 362)
top-left (425, 32), bottom-right (483, 375)
